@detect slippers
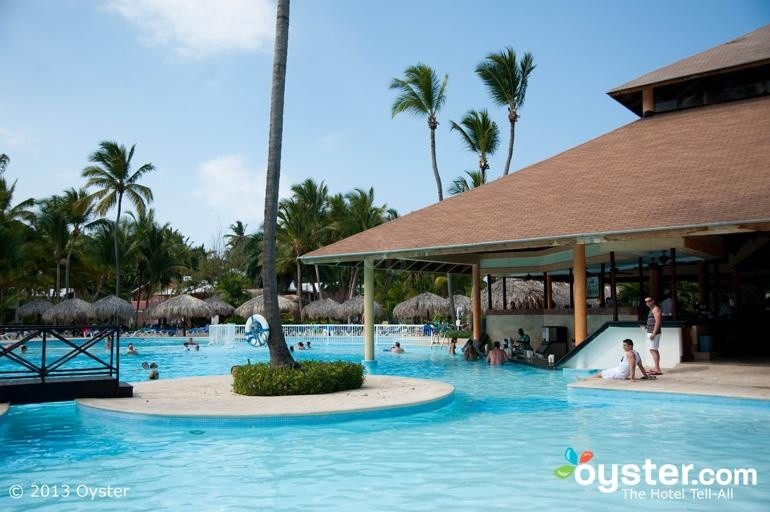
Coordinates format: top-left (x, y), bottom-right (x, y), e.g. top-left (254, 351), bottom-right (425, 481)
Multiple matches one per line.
top-left (646, 369), bottom-right (663, 375)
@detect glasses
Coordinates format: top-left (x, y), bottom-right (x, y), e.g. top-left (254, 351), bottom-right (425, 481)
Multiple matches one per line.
top-left (645, 300), bottom-right (652, 303)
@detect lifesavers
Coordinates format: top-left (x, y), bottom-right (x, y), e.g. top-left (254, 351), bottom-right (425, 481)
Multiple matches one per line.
top-left (244, 314), bottom-right (270, 347)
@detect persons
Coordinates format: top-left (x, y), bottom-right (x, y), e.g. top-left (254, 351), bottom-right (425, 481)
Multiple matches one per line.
top-left (18, 345), bottom-right (27, 354)
top-left (127, 343), bottom-right (160, 381)
top-left (289, 342), bottom-right (311, 352)
top-left (448, 337), bottom-right (457, 355)
top-left (576, 341), bottom-right (656, 382)
top-left (658, 292), bottom-right (673, 315)
top-left (390, 342), bottom-right (404, 354)
top-left (184, 337), bottom-right (199, 352)
top-left (510, 301), bottom-right (516, 310)
top-left (465, 328), bottom-right (534, 369)
top-left (391, 347), bottom-right (395, 351)
top-left (645, 297), bottom-right (663, 376)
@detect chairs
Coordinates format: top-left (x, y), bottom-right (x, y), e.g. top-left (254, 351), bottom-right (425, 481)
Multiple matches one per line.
top-left (0, 324), bottom-right (419, 342)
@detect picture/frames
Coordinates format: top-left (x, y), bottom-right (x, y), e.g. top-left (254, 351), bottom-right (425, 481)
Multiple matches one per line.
top-left (585, 274), bottom-right (602, 300)
